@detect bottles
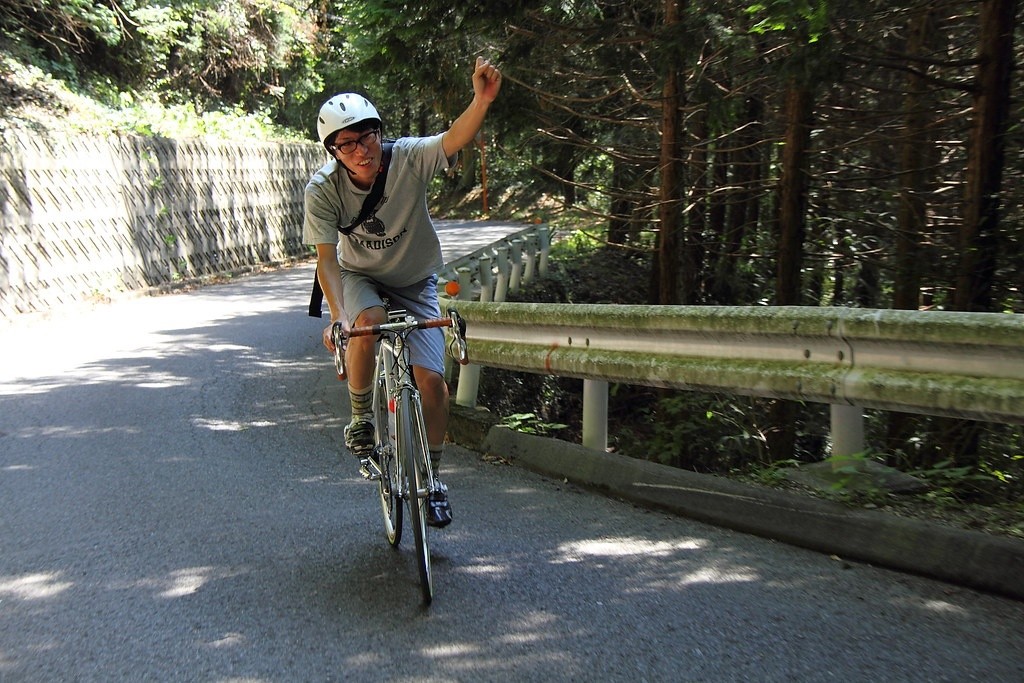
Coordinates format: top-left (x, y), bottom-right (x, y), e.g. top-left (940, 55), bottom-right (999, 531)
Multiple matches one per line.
top-left (388, 400), bottom-right (395, 449)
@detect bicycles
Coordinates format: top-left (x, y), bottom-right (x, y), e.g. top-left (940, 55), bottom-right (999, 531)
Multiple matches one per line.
top-left (330, 290), bottom-right (471, 606)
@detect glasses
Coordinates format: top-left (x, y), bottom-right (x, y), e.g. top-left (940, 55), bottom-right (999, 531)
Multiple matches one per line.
top-left (332, 130), bottom-right (379, 155)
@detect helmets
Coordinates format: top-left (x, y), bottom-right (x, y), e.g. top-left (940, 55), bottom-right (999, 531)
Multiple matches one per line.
top-left (317, 93), bottom-right (382, 154)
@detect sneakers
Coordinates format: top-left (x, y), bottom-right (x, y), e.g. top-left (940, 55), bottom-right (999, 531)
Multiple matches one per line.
top-left (421, 474), bottom-right (452, 526)
top-left (344, 420), bottom-right (376, 452)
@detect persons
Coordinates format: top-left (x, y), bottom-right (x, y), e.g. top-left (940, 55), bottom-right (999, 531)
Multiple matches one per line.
top-left (302, 56), bottom-right (502, 528)
